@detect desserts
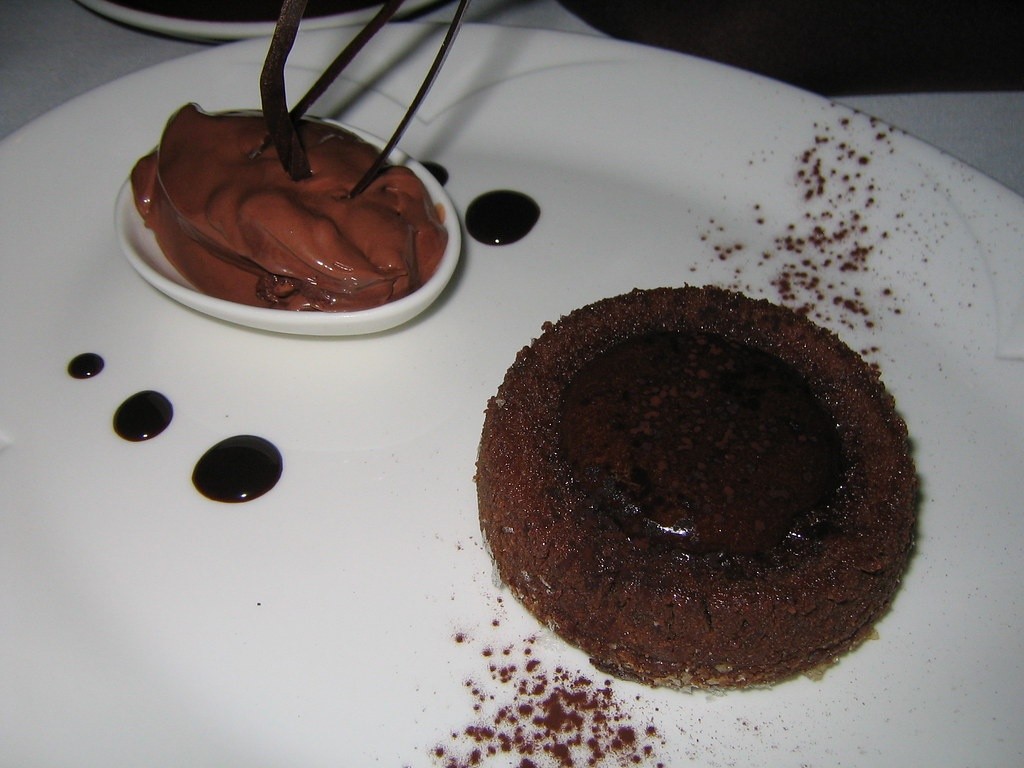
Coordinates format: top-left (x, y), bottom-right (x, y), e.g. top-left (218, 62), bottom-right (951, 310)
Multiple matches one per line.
top-left (477, 282), bottom-right (921, 692)
top-left (129, 0), bottom-right (472, 313)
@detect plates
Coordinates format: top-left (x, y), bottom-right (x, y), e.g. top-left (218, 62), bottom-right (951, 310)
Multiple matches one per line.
top-left (115, 109), bottom-right (464, 340)
top-left (79, 1), bottom-right (441, 47)
top-left (1, 24), bottom-right (1024, 768)
